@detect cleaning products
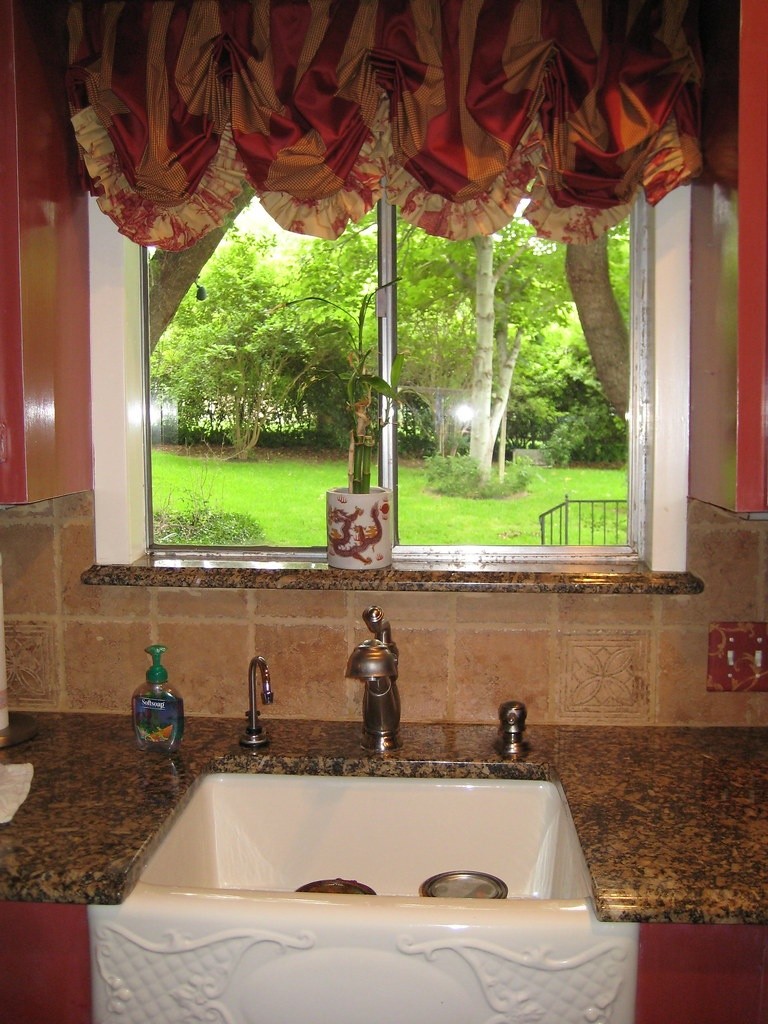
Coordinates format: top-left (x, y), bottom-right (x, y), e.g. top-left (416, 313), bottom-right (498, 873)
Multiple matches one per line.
top-left (131, 643), bottom-right (185, 754)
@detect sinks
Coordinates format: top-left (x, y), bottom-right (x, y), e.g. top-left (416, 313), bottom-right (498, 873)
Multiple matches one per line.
top-left (135, 769), bottom-right (594, 904)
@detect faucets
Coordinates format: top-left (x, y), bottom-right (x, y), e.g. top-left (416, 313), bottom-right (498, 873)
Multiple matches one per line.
top-left (342, 604), bottom-right (405, 755)
top-left (239, 655), bottom-right (274, 749)
